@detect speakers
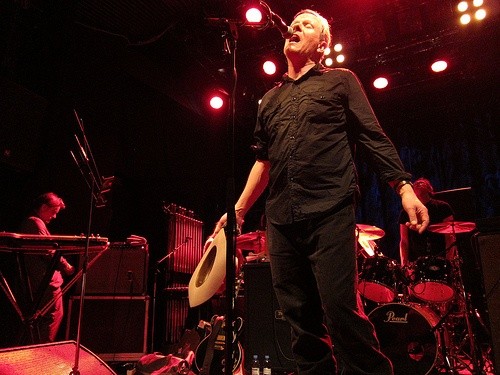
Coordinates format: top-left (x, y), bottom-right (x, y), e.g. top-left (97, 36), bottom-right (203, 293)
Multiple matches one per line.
top-left (74, 242), bottom-right (149, 296)
top-left (240, 262), bottom-right (299, 375)
top-left (0, 340), bottom-right (118, 375)
top-left (469, 233), bottom-right (500, 375)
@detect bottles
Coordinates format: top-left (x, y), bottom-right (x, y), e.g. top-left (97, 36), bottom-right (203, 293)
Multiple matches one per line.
top-left (262, 355), bottom-right (272, 375)
top-left (251, 356), bottom-right (261, 375)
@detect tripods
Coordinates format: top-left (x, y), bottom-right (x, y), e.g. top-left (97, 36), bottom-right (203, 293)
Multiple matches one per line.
top-left (449, 224), bottom-right (491, 372)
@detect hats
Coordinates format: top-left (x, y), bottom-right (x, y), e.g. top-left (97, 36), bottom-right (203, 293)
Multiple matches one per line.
top-left (188, 228), bottom-right (228, 308)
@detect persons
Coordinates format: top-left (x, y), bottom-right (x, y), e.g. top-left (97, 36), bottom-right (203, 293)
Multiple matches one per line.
top-left (398, 178), bottom-right (458, 294)
top-left (21, 192), bottom-right (75, 343)
top-left (212, 9), bottom-right (430, 375)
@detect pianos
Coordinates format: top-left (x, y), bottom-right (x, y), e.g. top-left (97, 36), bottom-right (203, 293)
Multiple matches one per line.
top-left (0, 230), bottom-right (110, 347)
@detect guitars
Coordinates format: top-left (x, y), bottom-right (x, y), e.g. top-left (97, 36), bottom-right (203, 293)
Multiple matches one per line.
top-left (194, 271), bottom-right (244, 375)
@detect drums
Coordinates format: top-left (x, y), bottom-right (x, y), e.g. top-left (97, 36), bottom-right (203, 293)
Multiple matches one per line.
top-left (368, 302), bottom-right (451, 375)
top-left (409, 254), bottom-right (459, 303)
top-left (357, 255), bottom-right (401, 304)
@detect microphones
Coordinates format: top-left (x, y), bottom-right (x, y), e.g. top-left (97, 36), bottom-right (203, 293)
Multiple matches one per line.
top-left (127, 270), bottom-right (131, 282)
top-left (270, 12), bottom-right (294, 39)
top-left (409, 346), bottom-right (423, 354)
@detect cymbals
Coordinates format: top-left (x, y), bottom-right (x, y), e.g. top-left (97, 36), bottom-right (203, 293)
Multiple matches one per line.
top-left (355, 223), bottom-right (386, 241)
top-left (427, 221), bottom-right (477, 234)
